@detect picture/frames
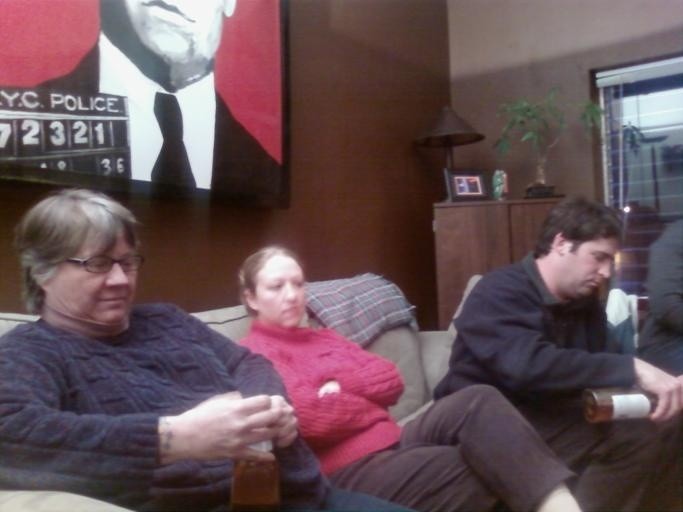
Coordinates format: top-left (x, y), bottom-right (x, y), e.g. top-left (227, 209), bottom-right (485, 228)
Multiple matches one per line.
top-left (444, 169), bottom-right (488, 201)
top-left (0, 0), bottom-right (292, 211)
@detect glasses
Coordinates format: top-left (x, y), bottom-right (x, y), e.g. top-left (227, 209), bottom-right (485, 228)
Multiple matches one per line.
top-left (58, 251), bottom-right (146, 274)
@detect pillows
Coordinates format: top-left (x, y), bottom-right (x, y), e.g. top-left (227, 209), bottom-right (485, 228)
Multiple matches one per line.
top-left (300, 277), bottom-right (425, 416)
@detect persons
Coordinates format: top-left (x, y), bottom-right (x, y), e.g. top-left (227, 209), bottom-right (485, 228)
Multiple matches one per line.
top-left (237, 245), bottom-right (582, 512)
top-left (36, 0), bottom-right (283, 189)
top-left (434, 195), bottom-right (683, 512)
top-left (0, 190), bottom-right (418, 512)
top-left (637, 218), bottom-right (683, 377)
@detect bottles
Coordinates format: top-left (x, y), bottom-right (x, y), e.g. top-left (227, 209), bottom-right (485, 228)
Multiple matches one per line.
top-left (233, 426), bottom-right (278, 512)
top-left (581, 386), bottom-right (659, 425)
top-left (492, 170), bottom-right (508, 201)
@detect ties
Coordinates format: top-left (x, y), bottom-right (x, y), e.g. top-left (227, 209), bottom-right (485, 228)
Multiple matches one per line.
top-left (150, 91), bottom-right (197, 189)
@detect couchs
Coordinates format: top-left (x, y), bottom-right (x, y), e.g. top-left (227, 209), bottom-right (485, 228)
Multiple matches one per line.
top-left (0, 273), bottom-right (482, 512)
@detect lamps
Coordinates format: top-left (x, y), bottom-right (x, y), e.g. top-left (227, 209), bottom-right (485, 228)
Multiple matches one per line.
top-left (414, 105), bottom-right (485, 168)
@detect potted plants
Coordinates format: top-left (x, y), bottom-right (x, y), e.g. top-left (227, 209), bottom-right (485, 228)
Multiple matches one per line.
top-left (489, 96), bottom-right (605, 197)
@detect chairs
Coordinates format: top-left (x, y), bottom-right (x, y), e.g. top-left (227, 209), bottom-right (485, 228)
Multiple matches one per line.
top-left (605, 289), bottom-right (649, 347)
